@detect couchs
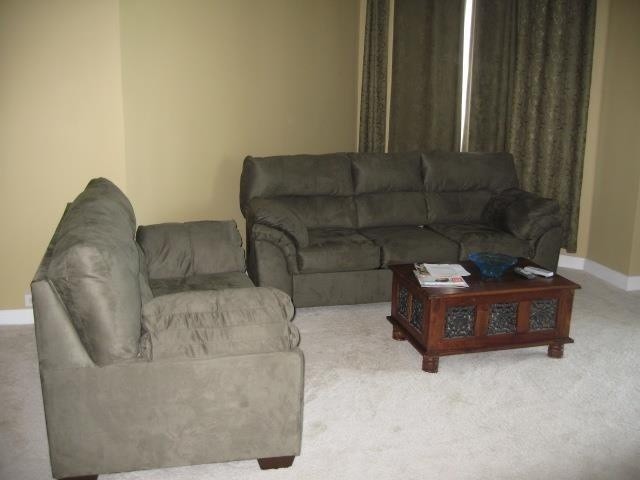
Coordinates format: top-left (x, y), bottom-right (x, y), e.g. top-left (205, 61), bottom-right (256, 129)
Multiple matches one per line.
top-left (240, 150), bottom-right (563, 308)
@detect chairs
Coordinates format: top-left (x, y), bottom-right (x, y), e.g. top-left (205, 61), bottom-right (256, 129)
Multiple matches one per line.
top-left (30, 178), bottom-right (305, 480)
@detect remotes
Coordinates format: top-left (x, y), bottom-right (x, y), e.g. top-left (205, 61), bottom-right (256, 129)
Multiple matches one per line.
top-left (524, 266), bottom-right (554, 278)
top-left (513, 267), bottom-right (537, 280)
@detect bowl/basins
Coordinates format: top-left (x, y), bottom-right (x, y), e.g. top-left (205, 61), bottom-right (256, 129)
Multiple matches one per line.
top-left (468, 252), bottom-right (518, 278)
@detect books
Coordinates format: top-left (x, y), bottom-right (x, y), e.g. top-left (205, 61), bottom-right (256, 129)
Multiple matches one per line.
top-left (413, 262), bottom-right (471, 290)
